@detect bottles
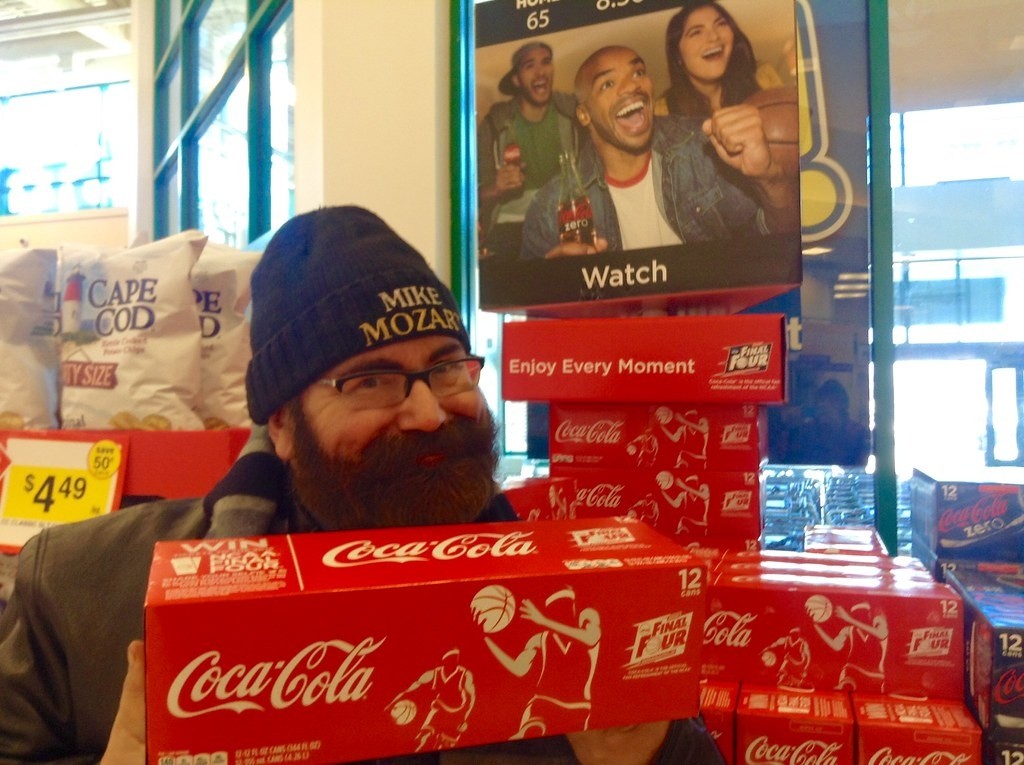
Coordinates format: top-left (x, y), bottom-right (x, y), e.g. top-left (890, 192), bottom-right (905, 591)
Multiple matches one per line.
top-left (503, 128), bottom-right (522, 198)
top-left (557, 150), bottom-right (598, 249)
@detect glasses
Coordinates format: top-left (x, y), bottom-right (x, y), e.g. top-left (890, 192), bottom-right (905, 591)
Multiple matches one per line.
top-left (317, 357), bottom-right (486, 410)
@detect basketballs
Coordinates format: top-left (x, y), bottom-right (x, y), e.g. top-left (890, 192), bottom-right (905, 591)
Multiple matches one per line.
top-left (741, 86), bottom-right (800, 179)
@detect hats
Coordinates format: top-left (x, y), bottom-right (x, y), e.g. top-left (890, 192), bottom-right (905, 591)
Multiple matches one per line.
top-left (246, 205), bottom-right (472, 426)
top-left (499, 42), bottom-right (553, 95)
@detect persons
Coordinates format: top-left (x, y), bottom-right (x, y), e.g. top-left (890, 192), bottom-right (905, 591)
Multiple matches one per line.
top-left (477, 4), bottom-right (800, 265)
top-left (0, 205), bottom-right (730, 765)
